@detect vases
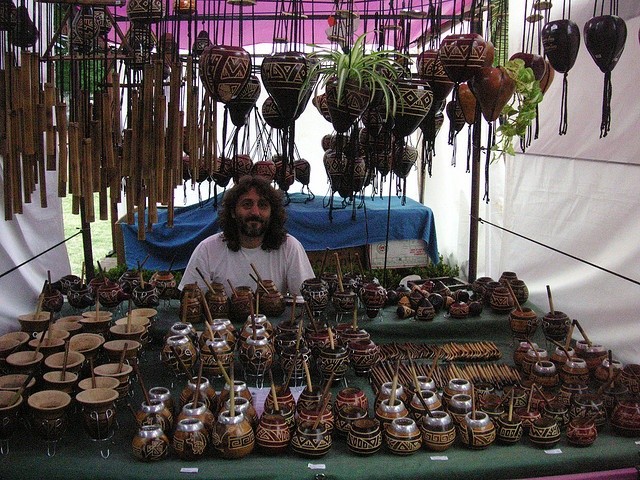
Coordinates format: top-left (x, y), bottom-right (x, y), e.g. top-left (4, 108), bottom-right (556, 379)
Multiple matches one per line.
top-left (260, 96), bottom-right (287, 129)
top-left (422, 110), bottom-right (444, 140)
top-left (583, 14), bottom-right (626, 138)
top-left (459, 86), bottom-right (480, 128)
top-left (181, 158), bottom-right (194, 178)
top-left (201, 45), bottom-right (251, 170)
top-left (191, 158), bottom-right (206, 181)
top-left (226, 75), bottom-right (259, 127)
top-left (392, 75), bottom-right (434, 135)
top-left (356, 127), bottom-right (392, 152)
top-left (313, 95), bottom-right (332, 123)
top-left (375, 153), bottom-right (392, 178)
top-left (349, 154), bottom-right (371, 196)
top-left (231, 153), bottom-right (252, 182)
top-left (418, 51), bottom-right (454, 103)
top-left (394, 147), bottom-right (420, 204)
top-left (439, 33), bottom-right (495, 85)
top-left (445, 101), bottom-right (466, 134)
top-left (124, 26), bottom-right (156, 69)
top-left (323, 150), bottom-right (346, 190)
top-left (321, 135), bottom-right (337, 145)
top-left (252, 160), bottom-right (275, 182)
top-left (293, 158), bottom-right (311, 187)
top-left (542, 20), bottom-right (577, 135)
top-left (275, 159), bottom-right (298, 190)
top-left (93, 2), bottom-right (113, 40)
top-left (70, 5), bottom-right (99, 49)
top-left (509, 52), bottom-right (552, 139)
top-left (210, 155), bottom-right (234, 189)
top-left (182, 124), bottom-right (201, 153)
top-left (127, 0), bottom-right (162, 24)
top-left (262, 52), bottom-right (318, 122)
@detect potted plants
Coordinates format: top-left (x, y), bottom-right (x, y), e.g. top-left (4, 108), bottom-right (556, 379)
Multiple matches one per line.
top-left (297, 33), bottom-right (412, 134)
top-left (469, 58), bottom-right (543, 161)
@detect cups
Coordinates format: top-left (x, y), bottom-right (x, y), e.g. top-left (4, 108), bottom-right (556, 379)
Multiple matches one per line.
top-left (44, 352), bottom-right (85, 372)
top-left (0, 374), bottom-right (37, 398)
top-left (69, 336), bottom-right (101, 353)
top-left (78, 376), bottom-right (121, 390)
top-left (0, 331), bottom-right (31, 355)
top-left (71, 333), bottom-right (105, 346)
top-left (50, 322), bottom-right (81, 331)
top-left (36, 330), bottom-right (70, 340)
top-left (126, 308), bottom-right (158, 318)
top-left (82, 310), bottom-right (113, 318)
top-left (43, 371), bottom-right (77, 393)
top-left (93, 363), bottom-right (133, 398)
top-left (77, 389), bottom-right (119, 439)
top-left (80, 317), bottom-right (111, 332)
top-left (5, 351), bottom-right (44, 375)
top-left (28, 389), bottom-right (71, 440)
top-left (103, 339), bottom-right (140, 375)
top-left (28, 338), bottom-right (65, 352)
top-left (114, 316), bottom-right (150, 326)
top-left (109, 324), bottom-right (145, 339)
top-left (0, 390), bottom-right (23, 438)
top-left (56, 316), bottom-right (84, 322)
top-left (19, 314), bottom-right (48, 329)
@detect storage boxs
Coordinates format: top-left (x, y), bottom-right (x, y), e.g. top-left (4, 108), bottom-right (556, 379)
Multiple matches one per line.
top-left (368, 198), bottom-right (430, 270)
top-left (302, 248), bottom-right (367, 276)
top-left (115, 207), bottom-right (145, 270)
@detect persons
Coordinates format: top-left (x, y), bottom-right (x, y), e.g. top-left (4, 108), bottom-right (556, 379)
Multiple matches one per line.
top-left (177, 174), bottom-right (317, 299)
